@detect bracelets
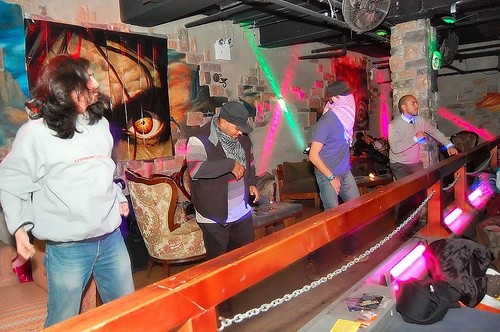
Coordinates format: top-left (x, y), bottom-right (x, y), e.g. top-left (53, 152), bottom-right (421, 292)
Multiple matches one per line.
top-left (327, 173), bottom-right (334, 180)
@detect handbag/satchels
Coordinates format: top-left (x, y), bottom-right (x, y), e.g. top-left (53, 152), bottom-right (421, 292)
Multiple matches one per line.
top-left (428, 237), bottom-right (491, 309)
top-left (394, 279), bottom-right (461, 324)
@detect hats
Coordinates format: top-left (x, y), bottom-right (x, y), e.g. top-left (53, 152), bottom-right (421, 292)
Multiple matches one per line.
top-left (324, 80), bottom-right (357, 97)
top-left (220, 102), bottom-right (253, 133)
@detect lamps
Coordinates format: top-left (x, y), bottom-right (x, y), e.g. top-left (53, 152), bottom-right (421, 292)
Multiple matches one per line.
top-left (214, 37), bottom-right (233, 61)
top-left (441, 0), bottom-right (464, 24)
top-left (303, 146), bottom-right (310, 155)
top-left (432, 51), bottom-right (443, 71)
top-left (374, 30), bottom-right (387, 36)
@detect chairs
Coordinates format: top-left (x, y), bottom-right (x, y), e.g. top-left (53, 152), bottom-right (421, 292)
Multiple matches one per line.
top-left (125, 156), bottom-right (277, 279)
top-left (450, 130), bottom-right (479, 151)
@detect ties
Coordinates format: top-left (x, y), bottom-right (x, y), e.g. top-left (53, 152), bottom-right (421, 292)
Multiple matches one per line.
top-left (410, 120), bottom-right (414, 125)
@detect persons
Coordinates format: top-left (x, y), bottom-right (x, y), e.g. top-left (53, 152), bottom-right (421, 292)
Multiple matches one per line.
top-left (186, 101), bottom-right (259, 324)
top-left (0, 54), bottom-right (136, 332)
top-left (305, 80), bottom-right (360, 280)
top-left (354, 131), bottom-right (390, 175)
top-left (388, 94), bottom-right (458, 227)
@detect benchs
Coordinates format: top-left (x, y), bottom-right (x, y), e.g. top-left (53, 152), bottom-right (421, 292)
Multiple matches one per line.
top-left (251, 203), bottom-right (303, 242)
top-left (355, 175), bottom-right (393, 196)
top-left (277, 159), bottom-right (321, 209)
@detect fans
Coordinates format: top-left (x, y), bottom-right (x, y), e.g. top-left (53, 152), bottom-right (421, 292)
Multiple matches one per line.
top-left (341, 0), bottom-right (391, 34)
